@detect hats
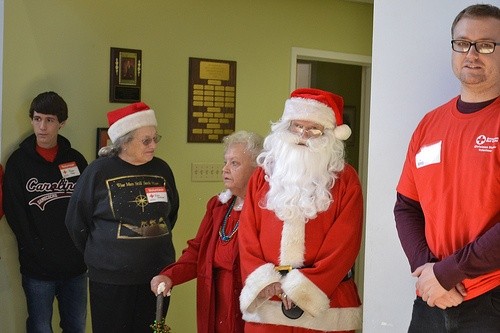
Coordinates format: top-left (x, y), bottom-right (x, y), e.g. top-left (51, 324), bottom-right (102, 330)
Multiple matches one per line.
top-left (282, 87), bottom-right (352, 141)
top-left (106, 102), bottom-right (157, 144)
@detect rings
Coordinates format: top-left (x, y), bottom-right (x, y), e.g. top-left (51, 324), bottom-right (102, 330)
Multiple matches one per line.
top-left (282, 294), bottom-right (288, 298)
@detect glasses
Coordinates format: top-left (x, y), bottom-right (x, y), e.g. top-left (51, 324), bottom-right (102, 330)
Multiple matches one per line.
top-left (142, 135), bottom-right (162, 146)
top-left (294, 126), bottom-right (324, 136)
top-left (451, 39), bottom-right (500, 54)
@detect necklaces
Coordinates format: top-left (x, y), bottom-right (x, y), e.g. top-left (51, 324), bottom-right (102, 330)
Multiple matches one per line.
top-left (219, 195), bottom-right (248, 242)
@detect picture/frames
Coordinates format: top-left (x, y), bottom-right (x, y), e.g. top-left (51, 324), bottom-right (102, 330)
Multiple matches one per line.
top-left (96, 127), bottom-right (108, 158)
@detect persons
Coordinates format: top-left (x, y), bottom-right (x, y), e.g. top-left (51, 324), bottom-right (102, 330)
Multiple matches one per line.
top-left (1, 91), bottom-right (87, 333)
top-left (393, 3), bottom-right (500, 333)
top-left (149, 129), bottom-right (265, 333)
top-left (237, 86), bottom-right (364, 333)
top-left (63, 102), bottom-right (179, 332)
